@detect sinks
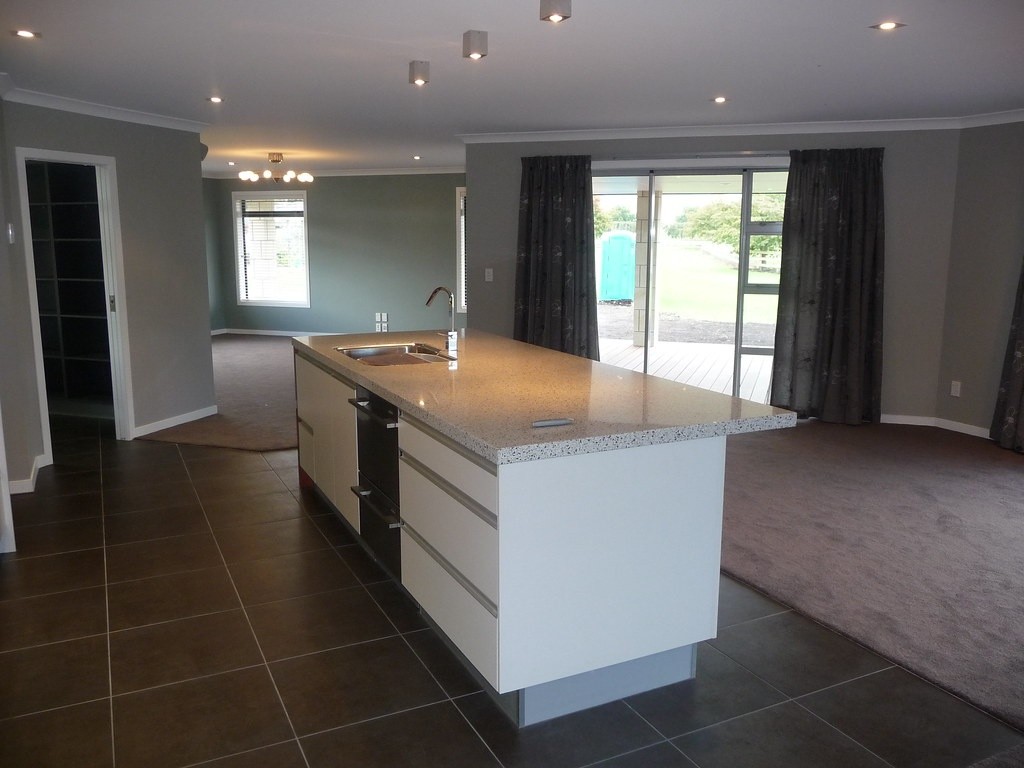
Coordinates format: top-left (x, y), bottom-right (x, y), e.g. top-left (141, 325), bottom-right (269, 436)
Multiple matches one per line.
top-left (333, 341), bottom-right (458, 367)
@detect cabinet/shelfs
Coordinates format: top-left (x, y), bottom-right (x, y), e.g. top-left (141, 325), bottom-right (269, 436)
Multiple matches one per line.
top-left (293, 348), bottom-right (726, 729)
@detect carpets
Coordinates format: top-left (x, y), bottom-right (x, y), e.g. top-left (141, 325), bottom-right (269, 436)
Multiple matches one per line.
top-left (719, 420), bottom-right (1023, 737)
top-left (134, 332), bottom-right (299, 452)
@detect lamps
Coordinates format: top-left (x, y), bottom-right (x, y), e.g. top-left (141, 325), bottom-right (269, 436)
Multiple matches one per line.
top-left (540, 0), bottom-right (572, 24)
top-left (462, 30), bottom-right (487, 60)
top-left (409, 60), bottom-right (430, 85)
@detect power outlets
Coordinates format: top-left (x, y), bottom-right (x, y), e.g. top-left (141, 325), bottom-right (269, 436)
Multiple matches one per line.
top-left (950, 381), bottom-right (961, 397)
top-left (485, 268), bottom-right (494, 282)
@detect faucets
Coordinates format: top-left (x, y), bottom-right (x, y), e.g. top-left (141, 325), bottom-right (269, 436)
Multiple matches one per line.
top-left (424, 283), bottom-right (458, 351)
